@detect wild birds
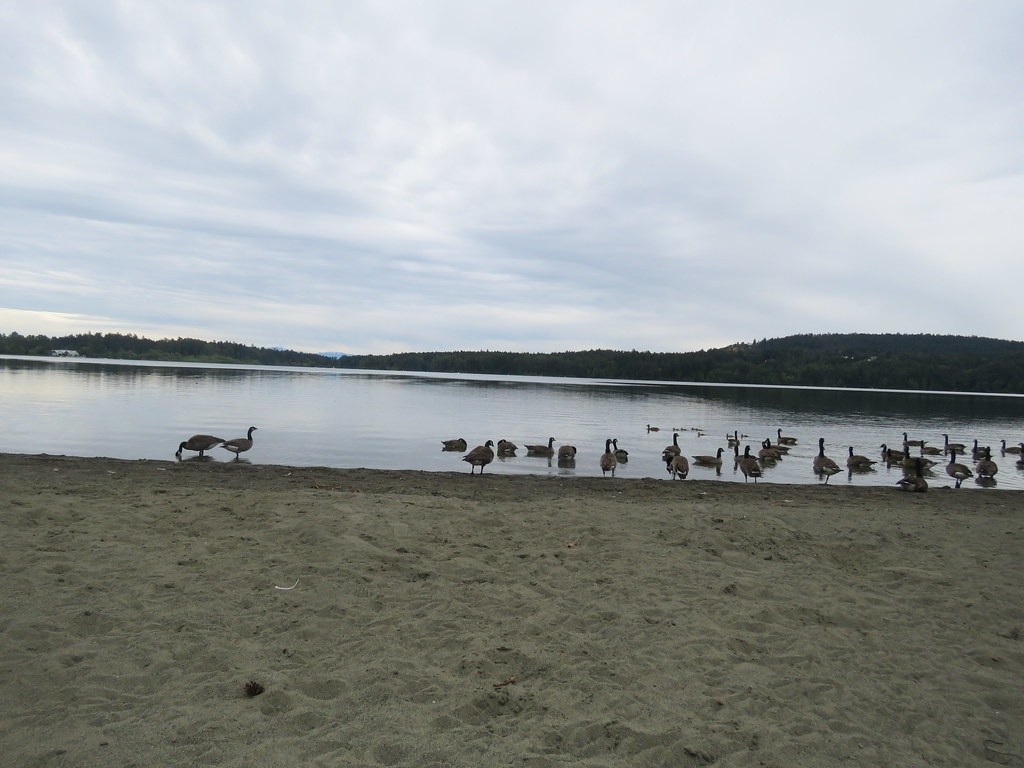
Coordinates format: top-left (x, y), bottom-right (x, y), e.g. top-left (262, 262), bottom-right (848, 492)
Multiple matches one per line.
top-left (673, 427), bottom-right (705, 438)
top-left (940, 434), bottom-right (975, 488)
top-left (847, 447), bottom-right (878, 467)
top-left (813, 438), bottom-right (844, 485)
top-left (175, 435), bottom-right (226, 462)
top-left (691, 447), bottom-right (725, 464)
top-left (441, 438), bottom-right (518, 475)
top-left (896, 459), bottom-right (928, 492)
top-left (971, 439), bottom-right (998, 479)
top-left (758, 429), bottom-right (798, 462)
top-left (1000, 439), bottom-right (1024, 459)
top-left (734, 445), bottom-right (763, 483)
top-left (524, 437), bottom-right (556, 454)
top-left (662, 433), bottom-right (689, 480)
top-left (646, 424), bottom-right (660, 431)
top-left (600, 438), bottom-right (628, 478)
top-left (727, 431), bottom-right (748, 448)
top-left (880, 432), bottom-right (943, 469)
top-left (219, 426), bottom-right (258, 462)
top-left (558, 446), bottom-right (577, 461)
